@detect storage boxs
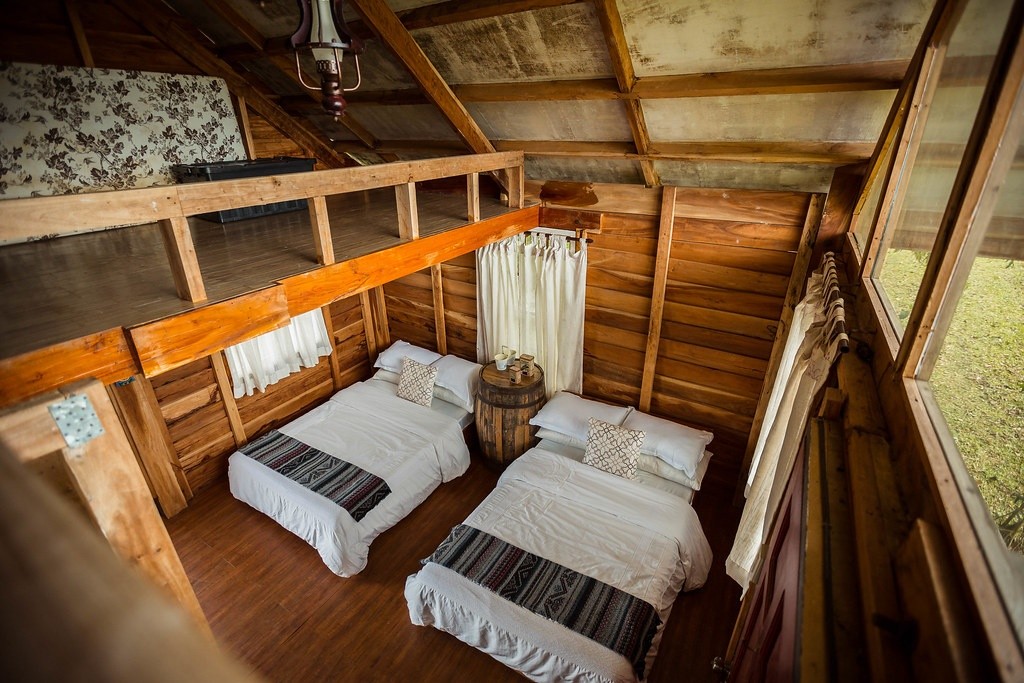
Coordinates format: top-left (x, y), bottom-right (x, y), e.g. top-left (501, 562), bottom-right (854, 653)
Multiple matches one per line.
top-left (172, 156), bottom-right (317, 223)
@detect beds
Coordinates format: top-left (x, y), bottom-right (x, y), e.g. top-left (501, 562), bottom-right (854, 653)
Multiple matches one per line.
top-left (404, 439), bottom-right (694, 683)
top-left (228, 377), bottom-right (475, 578)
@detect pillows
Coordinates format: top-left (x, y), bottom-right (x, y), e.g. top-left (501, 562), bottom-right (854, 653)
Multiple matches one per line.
top-left (373, 339), bottom-right (443, 375)
top-left (622, 406), bottom-right (714, 479)
top-left (638, 449), bottom-right (714, 491)
top-left (529, 391), bottom-right (632, 443)
top-left (396, 355), bottom-right (438, 407)
top-left (372, 368), bottom-right (400, 385)
top-left (581, 417), bottom-right (647, 481)
top-left (534, 427), bottom-right (587, 451)
top-left (433, 385), bottom-right (474, 414)
top-left (431, 354), bottom-right (484, 407)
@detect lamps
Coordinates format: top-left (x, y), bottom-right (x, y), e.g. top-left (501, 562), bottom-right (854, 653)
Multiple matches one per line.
top-left (290, 0), bottom-right (361, 122)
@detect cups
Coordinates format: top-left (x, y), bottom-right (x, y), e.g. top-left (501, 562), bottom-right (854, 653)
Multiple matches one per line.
top-left (505, 349), bottom-right (516, 365)
top-left (495, 353), bottom-right (508, 371)
